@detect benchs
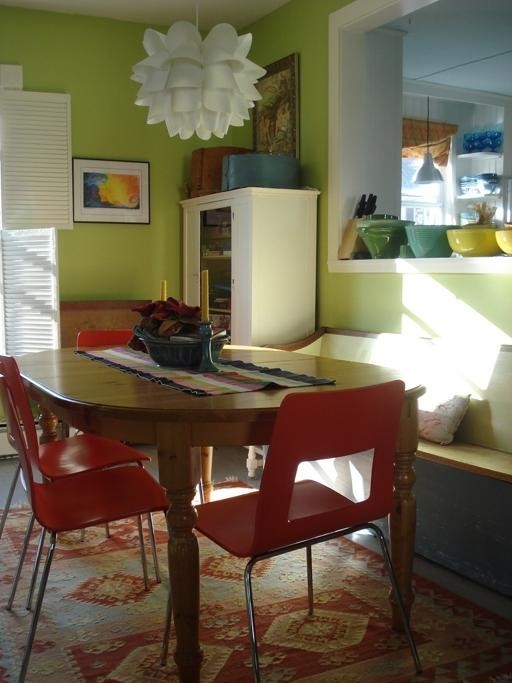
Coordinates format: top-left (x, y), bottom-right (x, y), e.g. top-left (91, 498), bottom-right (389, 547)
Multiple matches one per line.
top-left (261, 329), bottom-right (512, 596)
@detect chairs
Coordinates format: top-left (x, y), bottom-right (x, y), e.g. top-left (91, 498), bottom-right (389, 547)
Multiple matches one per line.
top-left (77, 327), bottom-right (134, 348)
top-left (1, 373), bottom-right (170, 682)
top-left (160, 379), bottom-right (422, 682)
top-left (2, 356), bottom-right (161, 612)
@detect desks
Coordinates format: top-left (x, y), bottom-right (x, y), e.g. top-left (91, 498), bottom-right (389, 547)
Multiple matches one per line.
top-left (13, 344), bottom-right (423, 680)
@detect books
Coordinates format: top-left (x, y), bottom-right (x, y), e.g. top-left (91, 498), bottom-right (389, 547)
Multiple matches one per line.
top-left (203, 225), bottom-right (231, 257)
top-left (213, 299), bottom-right (224, 302)
top-left (216, 297), bottom-right (229, 300)
top-left (208, 327), bottom-right (227, 340)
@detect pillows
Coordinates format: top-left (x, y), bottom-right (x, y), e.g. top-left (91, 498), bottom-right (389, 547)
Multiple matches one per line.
top-left (413, 394), bottom-right (471, 448)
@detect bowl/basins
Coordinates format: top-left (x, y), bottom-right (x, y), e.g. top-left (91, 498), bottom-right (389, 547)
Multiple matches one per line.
top-left (493, 229), bottom-right (512, 257)
top-left (443, 225), bottom-right (506, 257)
top-left (136, 332), bottom-right (231, 371)
top-left (353, 218), bottom-right (418, 259)
top-left (404, 224), bottom-right (460, 257)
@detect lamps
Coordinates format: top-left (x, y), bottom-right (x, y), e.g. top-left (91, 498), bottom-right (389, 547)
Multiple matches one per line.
top-left (409, 97), bottom-right (444, 187)
top-left (127, 5), bottom-right (268, 141)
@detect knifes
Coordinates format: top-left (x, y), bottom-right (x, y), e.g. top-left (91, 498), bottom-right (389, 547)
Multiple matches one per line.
top-left (354, 194), bottom-right (379, 218)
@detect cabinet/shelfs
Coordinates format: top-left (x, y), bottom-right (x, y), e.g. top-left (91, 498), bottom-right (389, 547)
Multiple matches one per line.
top-left (177, 184), bottom-right (323, 347)
top-left (446, 122), bottom-right (511, 227)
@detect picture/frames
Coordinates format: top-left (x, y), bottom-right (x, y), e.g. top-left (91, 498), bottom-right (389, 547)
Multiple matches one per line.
top-left (252, 52), bottom-right (301, 159)
top-left (71, 157), bottom-right (152, 227)
